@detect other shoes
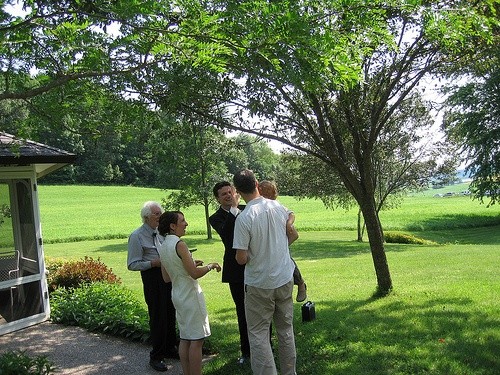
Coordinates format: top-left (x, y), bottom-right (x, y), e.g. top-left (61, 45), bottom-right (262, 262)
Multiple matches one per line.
top-left (296, 283), bottom-right (307, 302)
top-left (239, 356), bottom-right (247, 365)
top-left (149, 362), bottom-right (167, 370)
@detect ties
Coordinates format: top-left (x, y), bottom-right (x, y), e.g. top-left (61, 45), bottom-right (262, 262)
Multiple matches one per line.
top-left (152, 233), bottom-right (159, 256)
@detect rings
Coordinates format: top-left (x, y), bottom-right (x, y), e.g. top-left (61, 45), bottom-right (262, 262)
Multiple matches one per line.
top-left (216, 265), bottom-right (219, 267)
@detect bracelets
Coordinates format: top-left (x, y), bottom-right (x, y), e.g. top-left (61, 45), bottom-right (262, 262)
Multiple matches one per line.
top-left (205, 265), bottom-right (211, 271)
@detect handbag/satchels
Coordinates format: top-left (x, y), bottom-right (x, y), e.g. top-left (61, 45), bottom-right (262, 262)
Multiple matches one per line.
top-left (302, 301), bottom-right (316, 321)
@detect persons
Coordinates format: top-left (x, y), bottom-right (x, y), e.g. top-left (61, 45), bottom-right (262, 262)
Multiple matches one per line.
top-left (157, 210), bottom-right (221, 375)
top-left (126, 201), bottom-right (180, 372)
top-left (232, 169), bottom-right (299, 375)
top-left (258, 181), bottom-right (307, 301)
top-left (208, 181), bottom-right (273, 365)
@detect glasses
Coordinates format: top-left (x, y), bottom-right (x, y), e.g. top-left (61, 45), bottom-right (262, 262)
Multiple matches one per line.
top-left (150, 213), bottom-right (162, 217)
